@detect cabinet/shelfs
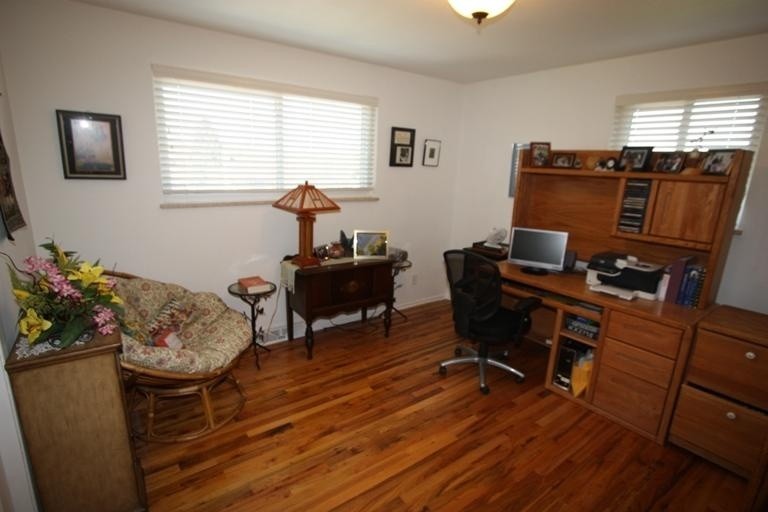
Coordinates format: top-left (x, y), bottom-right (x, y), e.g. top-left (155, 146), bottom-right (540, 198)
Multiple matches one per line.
top-left (670, 305), bottom-right (767, 499)
top-left (5, 322), bottom-right (147, 511)
top-left (511, 149), bottom-right (753, 254)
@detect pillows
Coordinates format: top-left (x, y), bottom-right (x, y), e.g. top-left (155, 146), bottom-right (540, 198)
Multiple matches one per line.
top-left (145, 299), bottom-right (195, 348)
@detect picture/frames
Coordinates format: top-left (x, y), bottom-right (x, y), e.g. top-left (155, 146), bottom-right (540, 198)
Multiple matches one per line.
top-left (530, 143), bottom-right (736, 175)
top-left (353, 229), bottom-right (389, 259)
top-left (56, 109), bottom-right (127, 180)
top-left (423, 139), bottom-right (441, 166)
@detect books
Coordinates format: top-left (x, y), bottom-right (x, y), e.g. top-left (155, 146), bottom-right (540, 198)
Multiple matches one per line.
top-left (297, 256), bottom-right (320, 269)
top-left (463, 240), bottom-right (511, 261)
top-left (619, 180), bottom-right (650, 233)
top-left (663, 256), bottom-right (708, 308)
top-left (237, 276), bottom-right (269, 294)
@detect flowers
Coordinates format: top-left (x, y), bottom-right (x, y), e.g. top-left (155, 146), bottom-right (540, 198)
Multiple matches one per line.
top-left (0, 237), bottom-right (124, 346)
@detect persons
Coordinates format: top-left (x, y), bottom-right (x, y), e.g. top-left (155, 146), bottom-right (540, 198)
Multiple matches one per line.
top-left (532, 144), bottom-right (735, 176)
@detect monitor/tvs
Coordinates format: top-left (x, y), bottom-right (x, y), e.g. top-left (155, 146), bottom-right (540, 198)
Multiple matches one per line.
top-left (508, 227), bottom-right (569, 275)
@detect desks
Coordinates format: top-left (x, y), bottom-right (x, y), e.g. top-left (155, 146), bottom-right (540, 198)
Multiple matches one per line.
top-left (281, 247), bottom-right (408, 360)
top-left (494, 257), bottom-right (719, 448)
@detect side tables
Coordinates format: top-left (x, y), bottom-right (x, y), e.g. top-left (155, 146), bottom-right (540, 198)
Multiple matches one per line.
top-left (228, 281), bottom-right (276, 370)
top-left (378, 260), bottom-right (412, 321)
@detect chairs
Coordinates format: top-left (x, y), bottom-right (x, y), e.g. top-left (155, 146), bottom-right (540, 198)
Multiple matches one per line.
top-left (438, 250), bottom-right (543, 395)
top-left (100, 267), bottom-right (247, 443)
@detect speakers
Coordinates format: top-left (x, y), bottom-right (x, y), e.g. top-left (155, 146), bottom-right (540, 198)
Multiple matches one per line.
top-left (565, 250), bottom-right (575, 274)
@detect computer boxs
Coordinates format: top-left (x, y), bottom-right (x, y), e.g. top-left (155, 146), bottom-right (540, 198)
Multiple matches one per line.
top-left (552, 343), bottom-right (586, 391)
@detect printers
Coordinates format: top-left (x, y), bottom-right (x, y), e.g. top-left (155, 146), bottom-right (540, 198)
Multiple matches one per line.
top-left (586, 253), bottom-right (672, 301)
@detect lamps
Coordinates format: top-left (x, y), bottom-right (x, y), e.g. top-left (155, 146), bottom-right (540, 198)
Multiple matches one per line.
top-left (271, 182), bottom-right (341, 269)
top-left (447, 0), bottom-right (515, 25)
